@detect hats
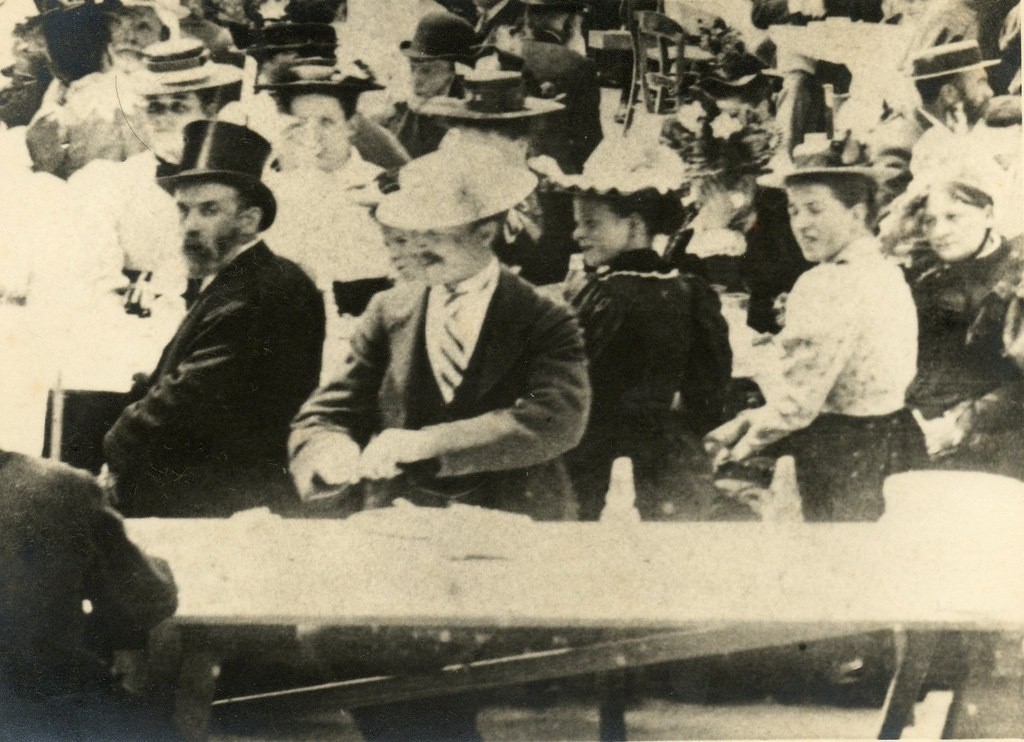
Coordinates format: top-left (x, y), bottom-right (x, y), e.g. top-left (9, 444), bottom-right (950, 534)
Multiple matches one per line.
top-left (754, 129), bottom-right (901, 191)
top-left (899, 37), bottom-right (1004, 82)
top-left (252, 50), bottom-right (387, 95)
top-left (406, 70), bottom-right (566, 122)
top-left (660, 99), bottom-right (779, 178)
top-left (696, 47), bottom-right (784, 96)
top-left (125, 37), bottom-right (244, 97)
top-left (532, 134), bottom-right (692, 200)
top-left (398, 13), bottom-right (493, 60)
top-left (16, 0), bottom-right (122, 35)
top-left (118, 3), bottom-right (190, 19)
top-left (893, 107), bottom-right (1024, 239)
top-left (156, 117), bottom-right (276, 232)
top-left (375, 146), bottom-right (536, 230)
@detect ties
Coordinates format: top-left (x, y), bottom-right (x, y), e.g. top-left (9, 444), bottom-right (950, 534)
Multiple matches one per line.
top-left (439, 283), bottom-right (477, 408)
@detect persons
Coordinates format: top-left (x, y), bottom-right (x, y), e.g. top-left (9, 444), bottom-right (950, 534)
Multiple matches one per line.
top-left (2, 440), bottom-right (178, 740)
top-left (2, 0), bottom-right (1023, 519)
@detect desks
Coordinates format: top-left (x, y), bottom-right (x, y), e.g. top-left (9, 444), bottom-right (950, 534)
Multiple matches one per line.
top-left (125, 520), bottom-right (1024, 742)
